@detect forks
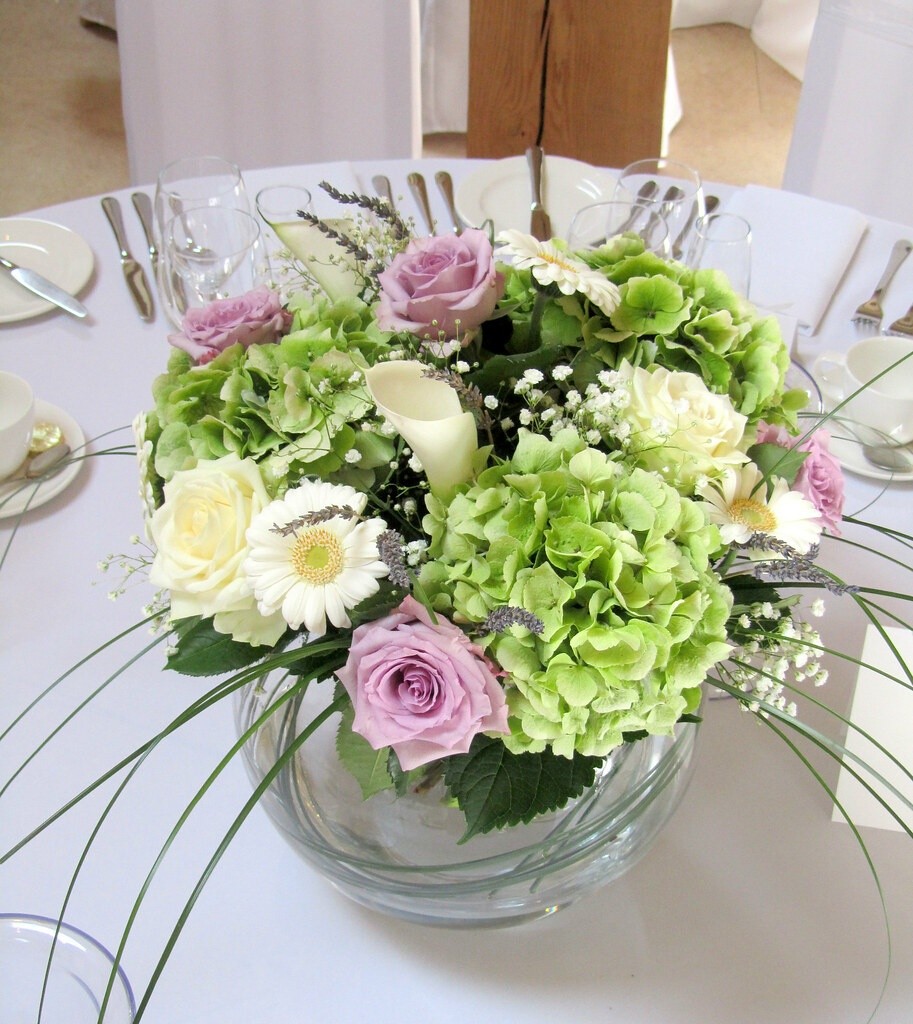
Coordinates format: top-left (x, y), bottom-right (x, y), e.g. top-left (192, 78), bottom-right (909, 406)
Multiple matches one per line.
top-left (887, 307), bottom-right (913, 336)
top-left (852, 240), bottom-right (911, 335)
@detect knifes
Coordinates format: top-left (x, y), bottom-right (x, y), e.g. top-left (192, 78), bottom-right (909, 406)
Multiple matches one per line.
top-left (528, 147), bottom-right (551, 242)
top-left (103, 198), bottom-right (154, 321)
top-left (0, 259), bottom-right (89, 319)
top-left (134, 193), bottom-right (188, 315)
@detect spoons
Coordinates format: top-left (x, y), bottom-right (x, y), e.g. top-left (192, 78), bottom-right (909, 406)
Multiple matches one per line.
top-left (0, 445), bottom-right (71, 484)
top-left (168, 193), bottom-right (219, 265)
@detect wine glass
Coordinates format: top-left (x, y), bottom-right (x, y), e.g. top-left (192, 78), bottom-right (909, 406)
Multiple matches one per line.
top-left (157, 209), bottom-right (260, 306)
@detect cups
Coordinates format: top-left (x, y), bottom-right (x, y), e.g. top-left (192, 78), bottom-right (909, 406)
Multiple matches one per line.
top-left (843, 338), bottom-right (913, 472)
top-left (609, 158), bottom-right (708, 278)
top-left (569, 202), bottom-right (672, 266)
top-left (0, 915), bottom-right (137, 1024)
top-left (0, 372), bottom-right (35, 484)
top-left (686, 213), bottom-right (753, 307)
top-left (154, 157), bottom-right (272, 332)
top-left (257, 187), bottom-right (315, 283)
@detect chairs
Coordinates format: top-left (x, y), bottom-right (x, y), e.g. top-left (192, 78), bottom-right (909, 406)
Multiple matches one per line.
top-left (114, 0), bottom-right (421, 186)
top-left (781, 0), bottom-right (913, 226)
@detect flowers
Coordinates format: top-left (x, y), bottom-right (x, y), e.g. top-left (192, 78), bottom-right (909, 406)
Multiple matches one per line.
top-left (2, 177), bottom-right (913, 1023)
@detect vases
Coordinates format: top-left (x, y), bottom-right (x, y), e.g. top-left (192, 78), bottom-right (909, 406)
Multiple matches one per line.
top-left (231, 629), bottom-right (705, 930)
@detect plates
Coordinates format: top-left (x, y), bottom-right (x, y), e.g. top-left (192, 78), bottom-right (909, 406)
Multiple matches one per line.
top-left (455, 155), bottom-right (631, 245)
top-left (791, 336), bottom-right (913, 479)
top-left (0, 219), bottom-right (95, 323)
top-left (0, 400), bottom-right (86, 520)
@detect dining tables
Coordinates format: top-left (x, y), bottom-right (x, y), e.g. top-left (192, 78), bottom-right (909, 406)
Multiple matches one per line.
top-left (1, 157), bottom-right (913, 1024)
